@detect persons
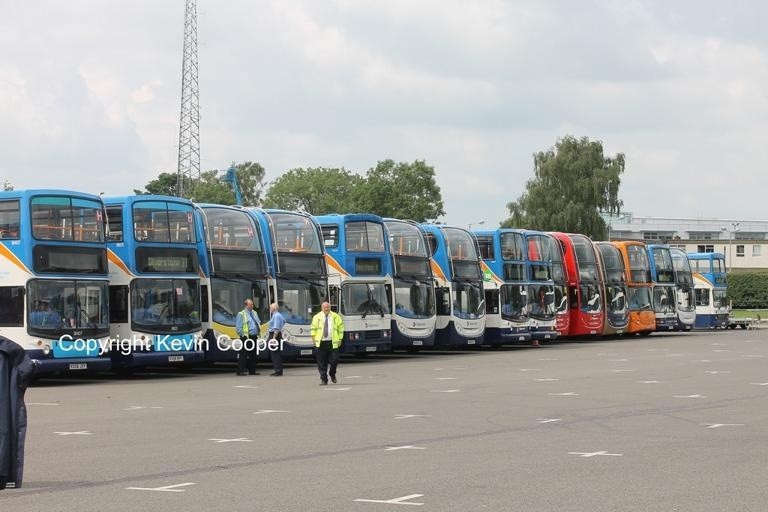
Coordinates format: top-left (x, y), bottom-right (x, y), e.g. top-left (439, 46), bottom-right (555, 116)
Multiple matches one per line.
top-left (235, 298), bottom-right (261, 376)
top-left (266, 302), bottom-right (285, 376)
top-left (310, 302), bottom-right (345, 386)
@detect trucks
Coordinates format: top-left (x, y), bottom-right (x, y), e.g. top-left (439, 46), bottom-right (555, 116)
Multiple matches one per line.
top-left (708, 298), bottom-right (758, 330)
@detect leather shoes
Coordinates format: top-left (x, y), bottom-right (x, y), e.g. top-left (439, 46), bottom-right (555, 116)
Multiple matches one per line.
top-left (250, 373), bottom-right (261, 375)
top-left (237, 373), bottom-right (248, 376)
top-left (321, 379), bottom-right (328, 385)
top-left (270, 372), bottom-right (283, 376)
top-left (329, 371), bottom-right (337, 383)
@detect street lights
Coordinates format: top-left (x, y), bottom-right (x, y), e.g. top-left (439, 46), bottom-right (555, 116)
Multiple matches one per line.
top-left (720, 222), bottom-right (740, 272)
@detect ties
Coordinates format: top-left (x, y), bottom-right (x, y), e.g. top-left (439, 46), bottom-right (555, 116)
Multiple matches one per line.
top-left (323, 315), bottom-right (329, 338)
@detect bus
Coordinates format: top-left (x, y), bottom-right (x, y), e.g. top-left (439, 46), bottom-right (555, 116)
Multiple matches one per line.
top-left (192, 202), bottom-right (696, 369)
top-left (0, 190), bottom-right (111, 386)
top-left (101, 195), bottom-right (205, 377)
top-left (685, 253), bottom-right (729, 330)
